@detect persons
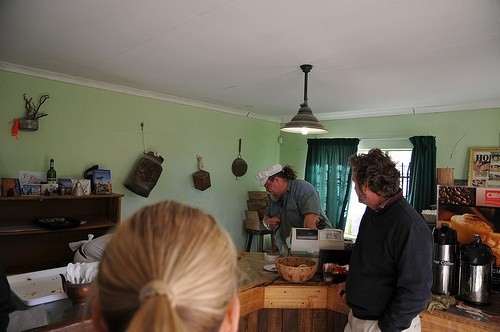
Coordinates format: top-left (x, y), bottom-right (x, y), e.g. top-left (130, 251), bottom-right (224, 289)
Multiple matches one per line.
top-left (256, 164), bottom-right (331, 248)
top-left (74, 232), bottom-right (114, 263)
top-left (343, 147), bottom-right (434, 332)
top-left (92, 201), bottom-right (240, 332)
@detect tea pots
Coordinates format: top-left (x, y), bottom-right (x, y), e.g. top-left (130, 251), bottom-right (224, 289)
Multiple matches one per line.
top-left (59, 273), bottom-right (96, 306)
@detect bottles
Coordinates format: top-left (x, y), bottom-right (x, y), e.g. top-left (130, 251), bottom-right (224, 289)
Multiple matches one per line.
top-left (47, 158), bottom-right (57, 184)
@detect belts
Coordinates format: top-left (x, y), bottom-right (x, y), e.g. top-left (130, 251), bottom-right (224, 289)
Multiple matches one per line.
top-left (79, 243), bottom-right (86, 259)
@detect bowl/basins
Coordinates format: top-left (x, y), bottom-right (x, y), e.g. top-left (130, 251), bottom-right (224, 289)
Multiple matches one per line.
top-left (276, 257), bottom-right (318, 283)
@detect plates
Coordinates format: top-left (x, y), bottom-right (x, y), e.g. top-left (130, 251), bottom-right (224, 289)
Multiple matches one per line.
top-left (34, 217), bottom-right (81, 231)
top-left (263, 264), bottom-right (277, 271)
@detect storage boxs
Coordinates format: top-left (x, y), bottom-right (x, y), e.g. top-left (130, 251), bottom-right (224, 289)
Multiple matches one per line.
top-left (92, 169), bottom-right (112, 195)
top-left (244, 191), bottom-right (268, 231)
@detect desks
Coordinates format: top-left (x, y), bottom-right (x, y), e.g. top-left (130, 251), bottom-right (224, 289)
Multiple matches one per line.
top-left (246, 229), bottom-right (277, 254)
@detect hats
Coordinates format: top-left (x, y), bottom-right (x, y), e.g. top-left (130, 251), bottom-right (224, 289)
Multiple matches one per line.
top-left (256, 164), bottom-right (282, 187)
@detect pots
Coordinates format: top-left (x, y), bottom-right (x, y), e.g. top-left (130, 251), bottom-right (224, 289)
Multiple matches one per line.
top-left (231, 138), bottom-right (247, 178)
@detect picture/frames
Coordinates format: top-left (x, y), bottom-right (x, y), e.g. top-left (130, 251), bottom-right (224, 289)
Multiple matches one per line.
top-left (2, 177), bottom-right (21, 196)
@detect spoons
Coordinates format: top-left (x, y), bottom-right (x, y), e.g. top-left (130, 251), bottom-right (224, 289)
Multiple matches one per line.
top-left (65, 262), bottom-right (98, 284)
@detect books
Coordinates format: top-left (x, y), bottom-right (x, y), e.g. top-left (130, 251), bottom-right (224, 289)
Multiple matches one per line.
top-left (93, 169), bottom-right (112, 195)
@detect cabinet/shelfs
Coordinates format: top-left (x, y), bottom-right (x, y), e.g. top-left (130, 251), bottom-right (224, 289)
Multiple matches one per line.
top-left (0, 192), bottom-right (124, 274)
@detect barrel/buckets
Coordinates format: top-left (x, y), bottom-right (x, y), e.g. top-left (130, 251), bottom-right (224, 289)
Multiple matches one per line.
top-left (457, 233), bottom-right (495, 307)
top-left (431, 223), bottom-right (459, 296)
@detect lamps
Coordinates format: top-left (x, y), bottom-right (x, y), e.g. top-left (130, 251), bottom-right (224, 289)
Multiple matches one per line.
top-left (280, 63), bottom-right (328, 136)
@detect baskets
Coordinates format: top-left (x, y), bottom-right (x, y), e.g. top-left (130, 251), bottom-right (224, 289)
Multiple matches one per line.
top-left (275, 253), bottom-right (318, 283)
